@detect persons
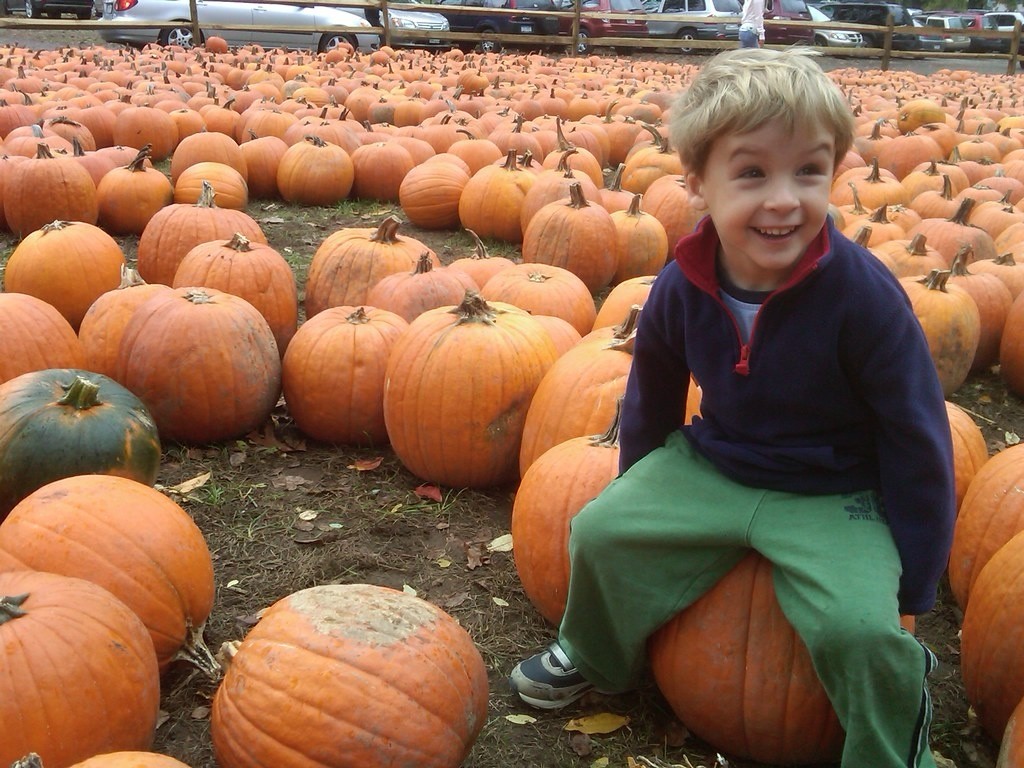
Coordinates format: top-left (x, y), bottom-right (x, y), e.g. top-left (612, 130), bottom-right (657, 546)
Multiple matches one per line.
top-left (738, 0), bottom-right (769, 48)
top-left (512, 48), bottom-right (956, 768)
top-left (364, 8), bottom-right (384, 28)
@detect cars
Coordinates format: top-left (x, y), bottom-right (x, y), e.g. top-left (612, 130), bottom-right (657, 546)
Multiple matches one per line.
top-left (0, 0), bottom-right (94, 19)
top-left (336, 0), bottom-right (451, 51)
top-left (806, 4), bottom-right (862, 58)
top-left (97, 0), bottom-right (381, 55)
top-left (912, 17), bottom-right (944, 59)
top-left (738, 0), bottom-right (814, 52)
top-left (640, 0), bottom-right (744, 54)
top-left (552, 0), bottom-right (648, 57)
top-left (432, 0), bottom-right (560, 55)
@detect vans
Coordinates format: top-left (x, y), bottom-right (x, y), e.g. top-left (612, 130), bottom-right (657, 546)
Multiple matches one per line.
top-left (817, 3), bottom-right (918, 59)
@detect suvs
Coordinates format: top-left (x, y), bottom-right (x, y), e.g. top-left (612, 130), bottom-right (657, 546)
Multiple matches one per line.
top-left (983, 12), bottom-right (1024, 52)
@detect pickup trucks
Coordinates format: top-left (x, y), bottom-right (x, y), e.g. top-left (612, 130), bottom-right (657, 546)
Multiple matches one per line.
top-left (911, 15), bottom-right (971, 56)
top-left (922, 11), bottom-right (1002, 53)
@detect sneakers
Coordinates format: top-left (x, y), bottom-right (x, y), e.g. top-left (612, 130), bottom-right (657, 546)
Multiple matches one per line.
top-left (509, 643), bottom-right (637, 710)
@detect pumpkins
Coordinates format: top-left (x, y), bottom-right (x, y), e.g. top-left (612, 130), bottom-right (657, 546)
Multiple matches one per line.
top-left (0, 37), bottom-right (1024, 768)
